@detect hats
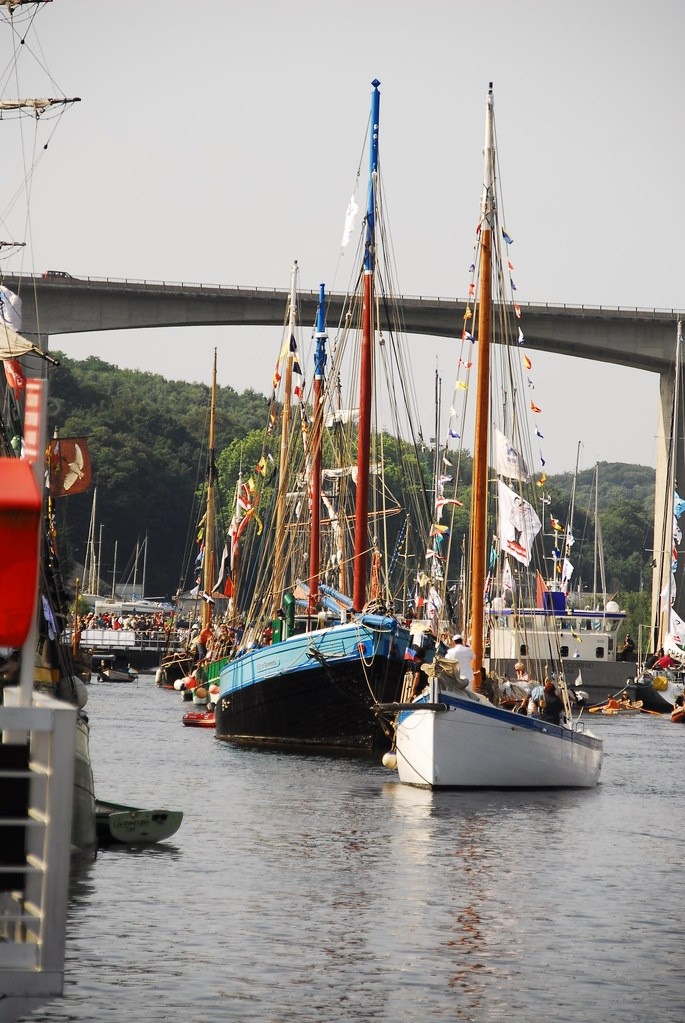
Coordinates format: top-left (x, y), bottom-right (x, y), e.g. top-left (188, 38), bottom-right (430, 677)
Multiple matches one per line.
top-left (452, 635), bottom-right (462, 641)
top-left (621, 691), bottom-right (628, 695)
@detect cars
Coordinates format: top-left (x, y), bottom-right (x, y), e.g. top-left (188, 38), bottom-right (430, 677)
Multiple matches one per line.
top-left (41, 271), bottom-right (79, 280)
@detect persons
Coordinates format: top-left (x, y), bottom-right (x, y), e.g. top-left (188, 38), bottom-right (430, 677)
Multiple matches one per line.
top-left (404, 607), bottom-right (417, 620)
top-left (78, 611), bottom-right (229, 645)
top-left (444, 634), bottom-right (476, 690)
top-left (198, 622), bottom-right (214, 661)
top-left (535, 684), bottom-right (564, 721)
top-left (652, 653), bottom-right (675, 669)
top-left (191, 624), bottom-right (199, 648)
top-left (607, 695), bottom-right (619, 709)
top-left (620, 692), bottom-right (632, 706)
top-left (224, 623), bottom-right (243, 648)
top-left (100, 659), bottom-right (109, 671)
top-left (408, 647), bottom-right (428, 701)
top-left (674, 688), bottom-right (685, 709)
top-left (620, 633), bottom-right (634, 661)
top-left (646, 652), bottom-right (659, 670)
top-left (421, 629), bottom-right (438, 665)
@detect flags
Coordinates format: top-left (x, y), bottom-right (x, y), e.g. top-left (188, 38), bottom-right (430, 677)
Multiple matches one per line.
top-left (499, 480), bottom-right (541, 566)
top-left (494, 427), bottom-right (527, 483)
top-left (670, 491), bottom-right (685, 606)
top-left (191, 183), bottom-right (590, 702)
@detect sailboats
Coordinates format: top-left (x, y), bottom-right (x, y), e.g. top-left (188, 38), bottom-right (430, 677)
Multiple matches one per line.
top-left (79, 489), bottom-right (105, 604)
top-left (1, 0), bottom-right (85, 646)
top-left (156, 82), bottom-right (448, 760)
top-left (625, 320), bottom-right (685, 714)
top-left (58, 578), bottom-right (94, 684)
top-left (374, 82), bottom-right (603, 793)
top-left (137, 537), bottom-right (173, 613)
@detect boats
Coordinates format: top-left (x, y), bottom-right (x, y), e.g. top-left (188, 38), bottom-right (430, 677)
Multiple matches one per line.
top-left (485, 550), bottom-right (636, 709)
top-left (96, 799), bottom-right (184, 844)
top-left (100, 666), bottom-right (138, 683)
top-left (59, 598), bottom-right (188, 670)
